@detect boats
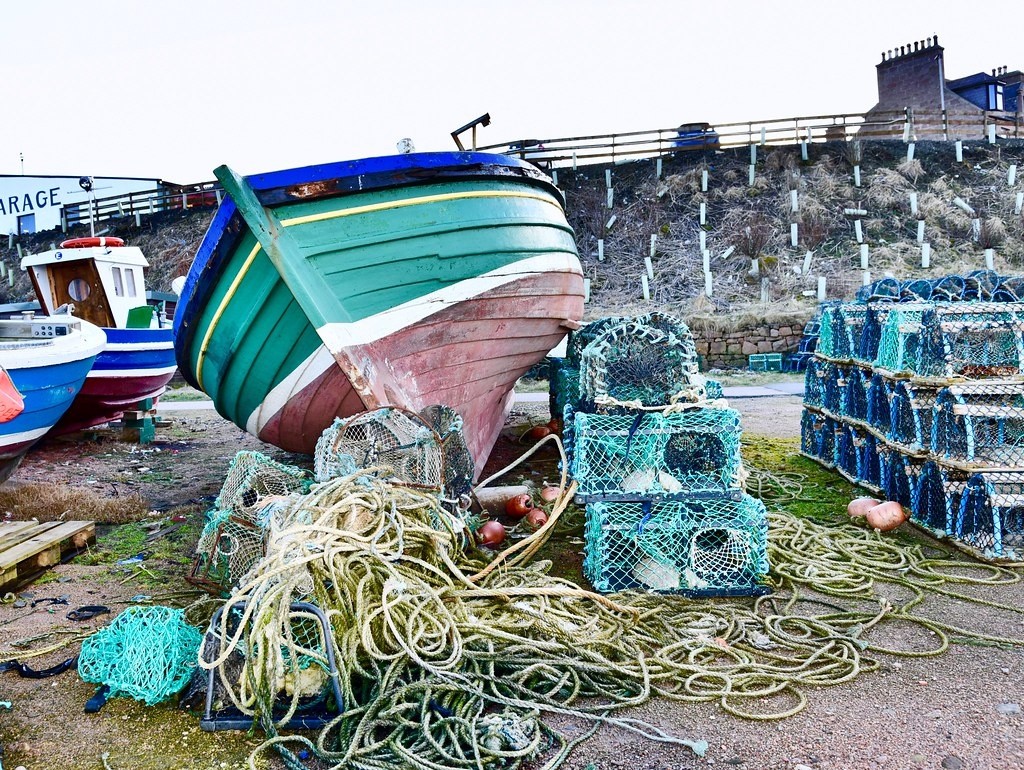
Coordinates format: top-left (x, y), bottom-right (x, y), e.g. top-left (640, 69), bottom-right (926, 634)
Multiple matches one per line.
top-left (173, 150), bottom-right (590, 491)
top-left (0, 175), bottom-right (179, 440)
top-left (0, 301), bottom-right (108, 484)
top-left (674, 122), bottom-right (720, 153)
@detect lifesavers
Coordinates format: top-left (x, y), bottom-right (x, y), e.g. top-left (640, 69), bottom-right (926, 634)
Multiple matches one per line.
top-left (60, 236), bottom-right (125, 248)
